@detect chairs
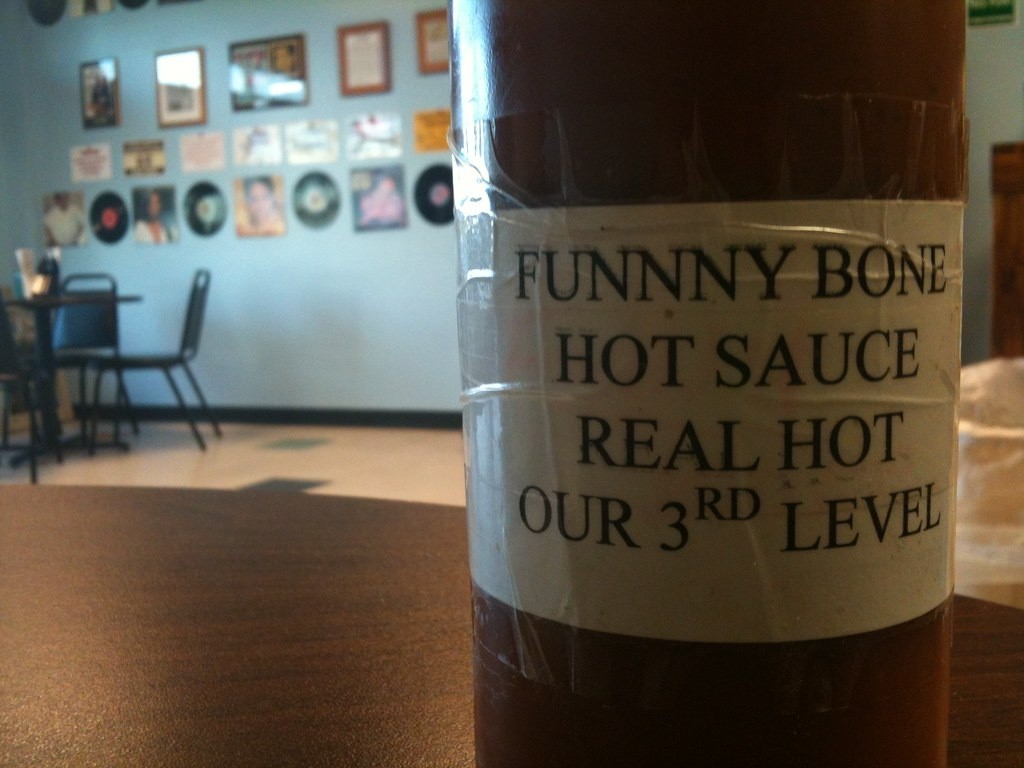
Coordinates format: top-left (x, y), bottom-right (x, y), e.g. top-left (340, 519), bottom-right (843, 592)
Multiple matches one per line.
top-left (0, 269), bottom-right (220, 485)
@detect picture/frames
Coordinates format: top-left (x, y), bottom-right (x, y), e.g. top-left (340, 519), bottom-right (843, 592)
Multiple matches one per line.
top-left (336, 20), bottom-right (391, 93)
top-left (153, 47), bottom-right (207, 128)
top-left (417, 8), bottom-right (450, 73)
top-left (80, 58), bottom-right (121, 128)
top-left (230, 33), bottom-right (312, 111)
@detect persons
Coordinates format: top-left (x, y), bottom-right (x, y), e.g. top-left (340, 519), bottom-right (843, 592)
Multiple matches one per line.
top-left (42, 191), bottom-right (86, 246)
top-left (238, 176), bottom-right (284, 234)
top-left (135, 189), bottom-right (177, 242)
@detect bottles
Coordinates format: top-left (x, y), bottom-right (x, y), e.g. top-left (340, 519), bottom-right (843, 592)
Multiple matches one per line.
top-left (447, 0), bottom-right (971, 768)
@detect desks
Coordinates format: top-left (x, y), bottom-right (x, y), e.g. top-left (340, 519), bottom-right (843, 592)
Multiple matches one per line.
top-left (0, 295), bottom-right (143, 461)
